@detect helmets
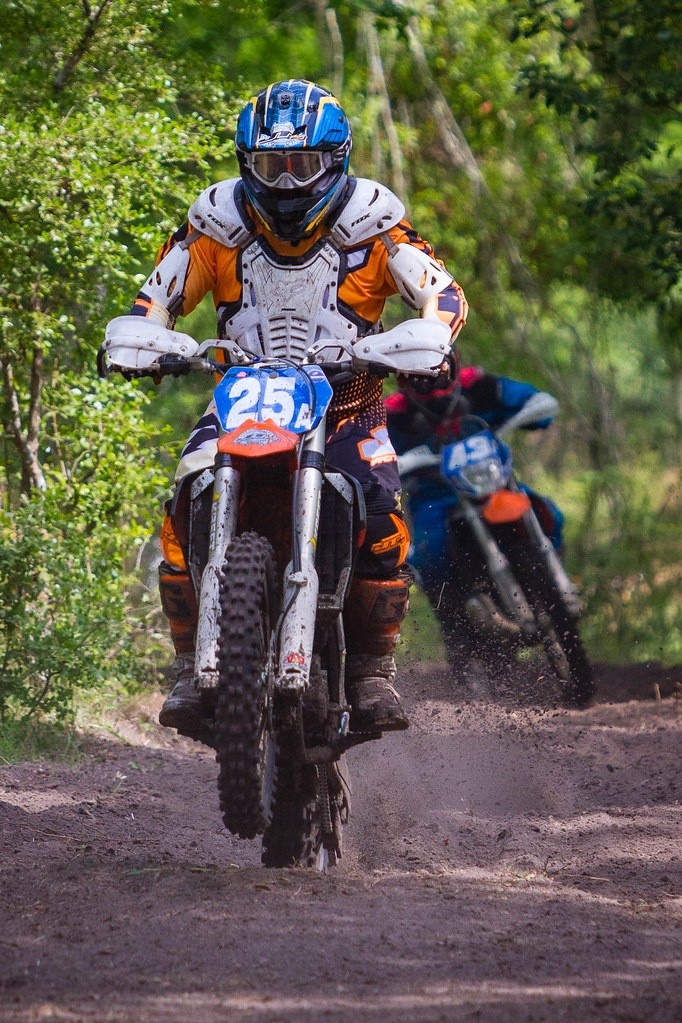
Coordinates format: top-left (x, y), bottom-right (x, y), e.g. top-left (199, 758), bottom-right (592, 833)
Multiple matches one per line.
top-left (394, 344), bottom-right (461, 394)
top-left (234, 78), bottom-right (353, 217)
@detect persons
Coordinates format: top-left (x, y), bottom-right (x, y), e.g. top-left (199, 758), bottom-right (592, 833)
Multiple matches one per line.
top-left (381, 343), bottom-right (567, 659)
top-left (121, 79), bottom-right (470, 731)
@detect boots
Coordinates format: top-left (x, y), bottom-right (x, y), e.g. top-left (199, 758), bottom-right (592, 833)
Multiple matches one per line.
top-left (342, 563), bottom-right (415, 731)
top-left (157, 560), bottom-right (212, 726)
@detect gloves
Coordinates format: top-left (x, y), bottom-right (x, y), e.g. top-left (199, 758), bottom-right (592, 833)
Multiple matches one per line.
top-left (119, 365), bottom-right (164, 386)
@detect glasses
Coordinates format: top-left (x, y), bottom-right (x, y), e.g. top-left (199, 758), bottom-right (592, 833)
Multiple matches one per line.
top-left (254, 153), bottom-right (322, 182)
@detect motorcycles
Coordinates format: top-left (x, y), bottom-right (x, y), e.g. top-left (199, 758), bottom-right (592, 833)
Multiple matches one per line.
top-left (101, 313), bottom-right (451, 871)
top-left (390, 392), bottom-right (600, 707)
